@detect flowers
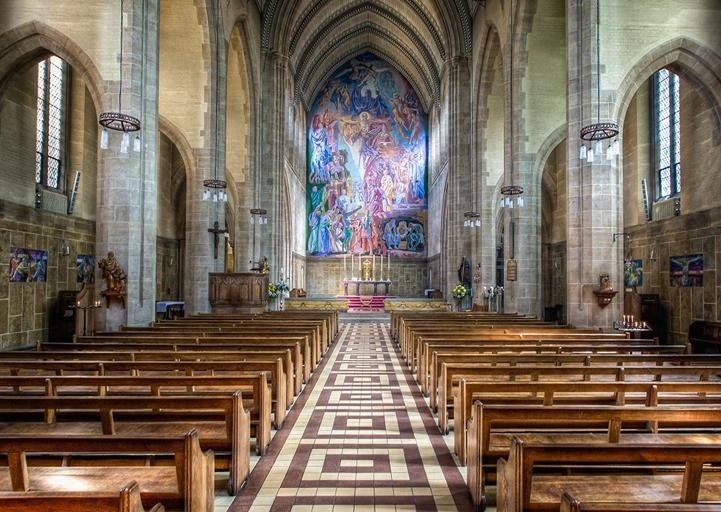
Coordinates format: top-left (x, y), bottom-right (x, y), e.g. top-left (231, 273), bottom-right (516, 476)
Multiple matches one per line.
top-left (266, 279), bottom-right (289, 301)
top-left (451, 284), bottom-right (466, 300)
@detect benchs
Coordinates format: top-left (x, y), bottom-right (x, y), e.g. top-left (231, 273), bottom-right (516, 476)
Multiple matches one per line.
top-left (388, 307), bottom-right (720, 510)
top-left (0, 307), bottom-right (340, 511)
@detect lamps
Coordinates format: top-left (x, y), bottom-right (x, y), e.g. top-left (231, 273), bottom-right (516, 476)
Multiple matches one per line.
top-left (578, 2), bottom-right (620, 164)
top-left (462, 1), bottom-right (523, 228)
top-left (97, 1), bottom-right (142, 154)
top-left (201, 1), bottom-right (267, 226)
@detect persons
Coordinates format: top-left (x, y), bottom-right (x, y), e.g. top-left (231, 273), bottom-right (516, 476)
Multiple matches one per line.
top-left (309, 65), bottom-right (424, 254)
